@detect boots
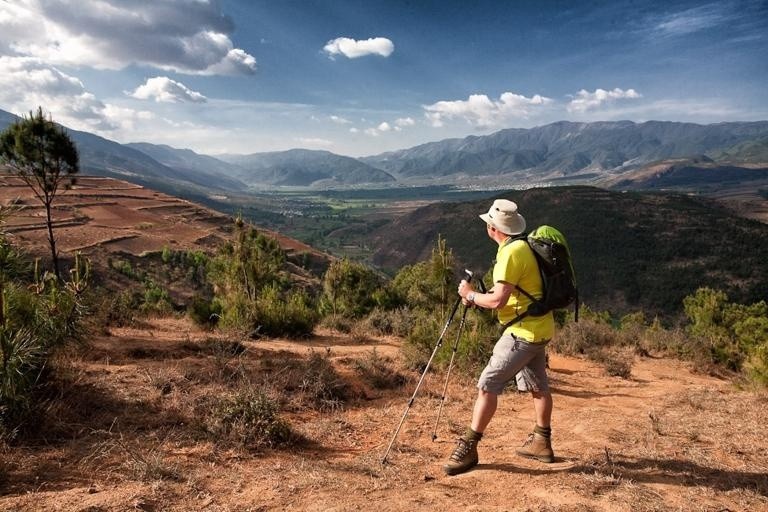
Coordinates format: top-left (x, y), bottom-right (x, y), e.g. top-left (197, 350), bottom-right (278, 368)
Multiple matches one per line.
top-left (444, 437), bottom-right (478, 475)
top-left (516, 431), bottom-right (554, 463)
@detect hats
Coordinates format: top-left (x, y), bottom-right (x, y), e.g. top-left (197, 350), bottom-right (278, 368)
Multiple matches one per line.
top-left (478, 199), bottom-right (527, 235)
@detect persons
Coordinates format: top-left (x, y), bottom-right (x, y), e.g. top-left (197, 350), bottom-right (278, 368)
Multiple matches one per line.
top-left (444, 199), bottom-right (554, 474)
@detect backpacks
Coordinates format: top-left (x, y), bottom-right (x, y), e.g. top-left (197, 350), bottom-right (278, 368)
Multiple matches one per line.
top-left (497, 225), bottom-right (577, 316)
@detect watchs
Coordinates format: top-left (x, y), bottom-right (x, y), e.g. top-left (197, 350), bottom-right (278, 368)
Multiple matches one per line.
top-left (466, 292), bottom-right (474, 303)
